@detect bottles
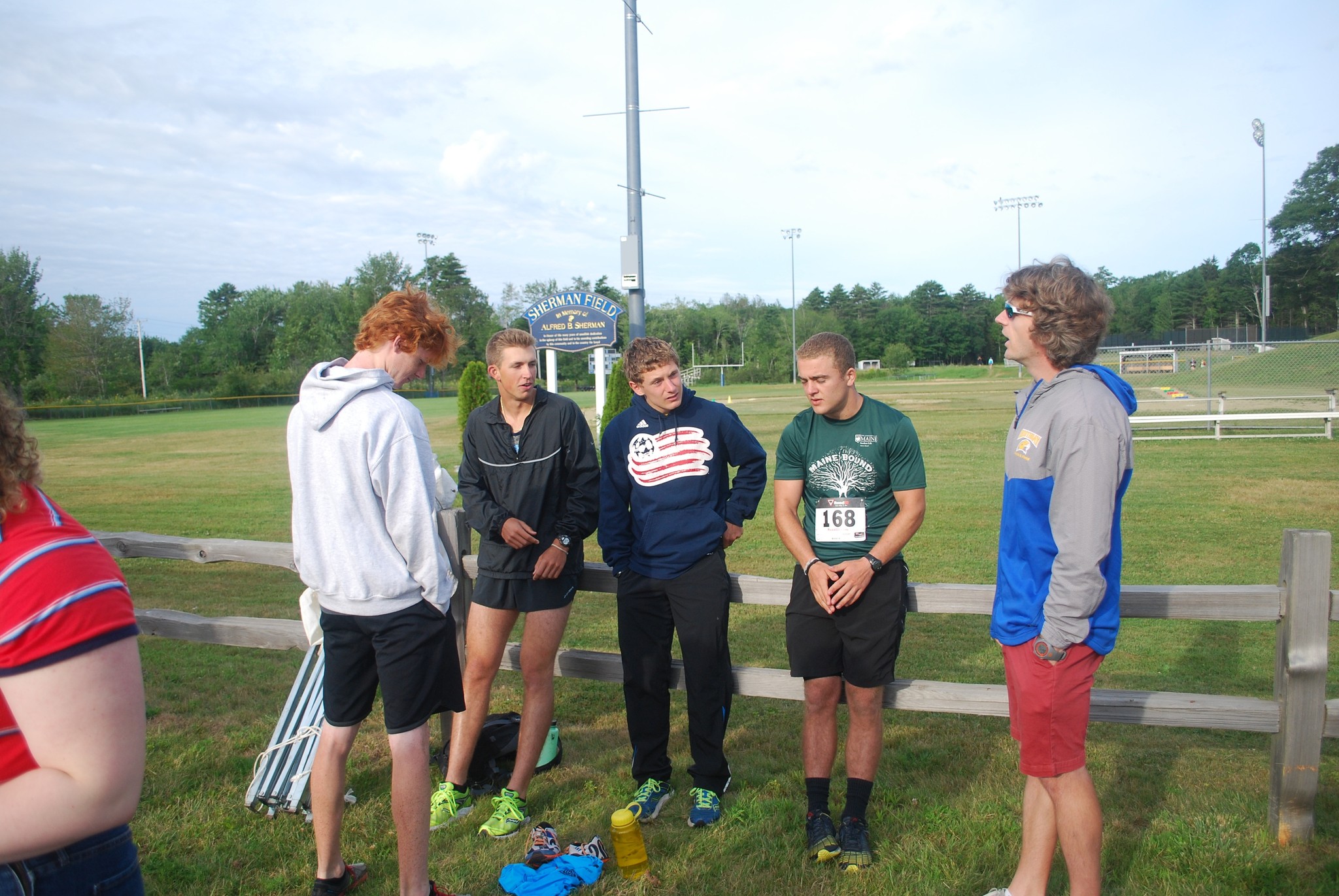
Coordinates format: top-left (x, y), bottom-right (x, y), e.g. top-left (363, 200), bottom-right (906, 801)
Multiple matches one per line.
top-left (610, 802), bottom-right (650, 880)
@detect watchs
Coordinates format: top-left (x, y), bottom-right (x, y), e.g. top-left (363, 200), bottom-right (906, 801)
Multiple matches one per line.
top-left (863, 553), bottom-right (883, 572)
top-left (1031, 636), bottom-right (1067, 661)
top-left (556, 533), bottom-right (573, 549)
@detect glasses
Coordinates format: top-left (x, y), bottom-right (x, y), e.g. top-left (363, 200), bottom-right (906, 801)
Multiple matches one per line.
top-left (1004, 300), bottom-right (1036, 318)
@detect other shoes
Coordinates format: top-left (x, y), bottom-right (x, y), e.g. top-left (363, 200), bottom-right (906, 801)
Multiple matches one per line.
top-left (981, 888), bottom-right (1013, 896)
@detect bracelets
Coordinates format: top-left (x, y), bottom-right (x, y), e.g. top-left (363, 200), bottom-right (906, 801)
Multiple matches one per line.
top-left (803, 557), bottom-right (820, 575)
top-left (551, 544), bottom-right (569, 554)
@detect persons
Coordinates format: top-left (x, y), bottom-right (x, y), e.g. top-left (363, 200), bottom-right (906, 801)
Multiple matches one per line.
top-left (976, 255), bottom-right (1137, 896)
top-left (596, 338), bottom-right (768, 827)
top-left (1, 381), bottom-right (148, 896)
top-left (284, 286), bottom-right (469, 896)
top-left (774, 332), bottom-right (928, 872)
top-left (427, 328), bottom-right (601, 840)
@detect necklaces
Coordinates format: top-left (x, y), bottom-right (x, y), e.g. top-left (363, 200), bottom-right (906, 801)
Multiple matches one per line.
top-left (499, 395), bottom-right (535, 431)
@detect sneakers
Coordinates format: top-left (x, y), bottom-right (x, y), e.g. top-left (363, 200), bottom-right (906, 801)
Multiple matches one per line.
top-left (628, 778), bottom-right (674, 823)
top-left (311, 859), bottom-right (368, 896)
top-left (687, 788), bottom-right (721, 828)
top-left (475, 788), bottom-right (531, 840)
top-left (429, 780), bottom-right (474, 832)
top-left (806, 813), bottom-right (841, 864)
top-left (525, 821), bottom-right (562, 869)
top-left (562, 834), bottom-right (610, 863)
top-left (428, 879), bottom-right (457, 896)
top-left (835, 818), bottom-right (872, 876)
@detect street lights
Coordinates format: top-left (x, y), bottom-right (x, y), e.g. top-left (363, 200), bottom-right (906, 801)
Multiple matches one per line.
top-left (781, 226), bottom-right (804, 386)
top-left (993, 193), bottom-right (1043, 272)
top-left (417, 231), bottom-right (441, 398)
top-left (1251, 119), bottom-right (1272, 355)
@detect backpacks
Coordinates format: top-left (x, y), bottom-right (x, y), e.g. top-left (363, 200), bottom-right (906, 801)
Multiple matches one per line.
top-left (433, 710), bottom-right (564, 792)
